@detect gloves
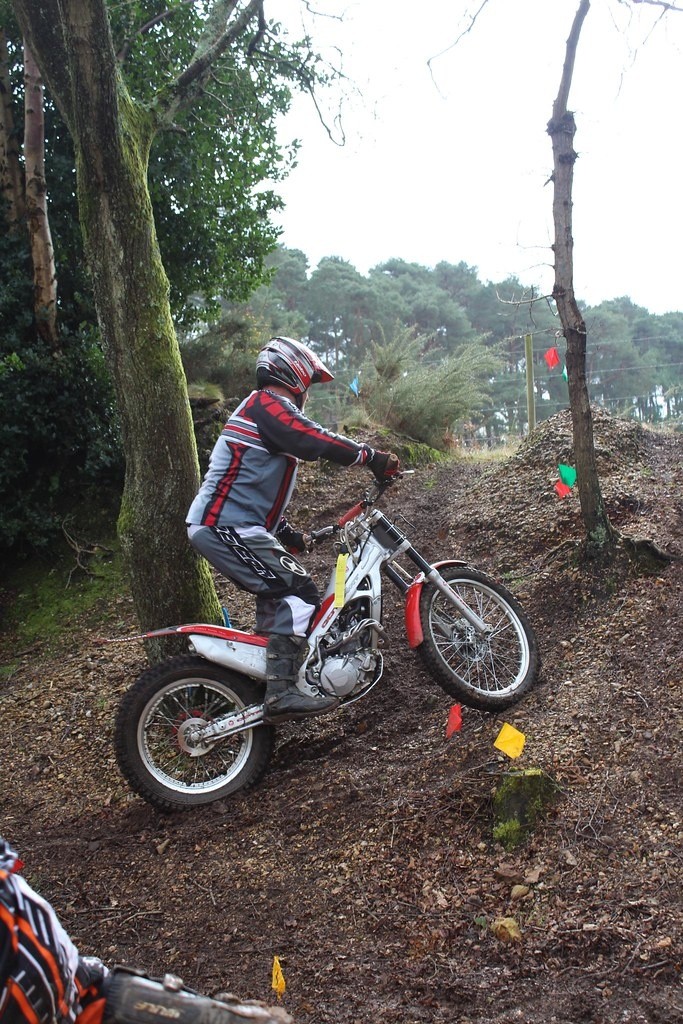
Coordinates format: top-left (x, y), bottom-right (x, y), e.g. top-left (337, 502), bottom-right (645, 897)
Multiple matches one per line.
top-left (277, 519), bottom-right (313, 557)
top-left (363, 443), bottom-right (403, 485)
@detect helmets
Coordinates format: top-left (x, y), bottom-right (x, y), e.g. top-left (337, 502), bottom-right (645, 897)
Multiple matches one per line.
top-left (256, 334), bottom-right (336, 405)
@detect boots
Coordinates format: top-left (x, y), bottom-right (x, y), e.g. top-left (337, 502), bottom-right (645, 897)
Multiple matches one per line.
top-left (260, 632), bottom-right (339, 724)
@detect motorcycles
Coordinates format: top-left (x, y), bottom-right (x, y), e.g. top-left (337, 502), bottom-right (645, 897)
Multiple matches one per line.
top-left (1, 931), bottom-right (300, 1024)
top-left (114, 454), bottom-right (544, 817)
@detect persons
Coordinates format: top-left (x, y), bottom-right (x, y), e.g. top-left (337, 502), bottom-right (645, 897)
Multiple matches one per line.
top-left (0, 833), bottom-right (109, 1024)
top-left (183, 334), bottom-right (401, 726)
